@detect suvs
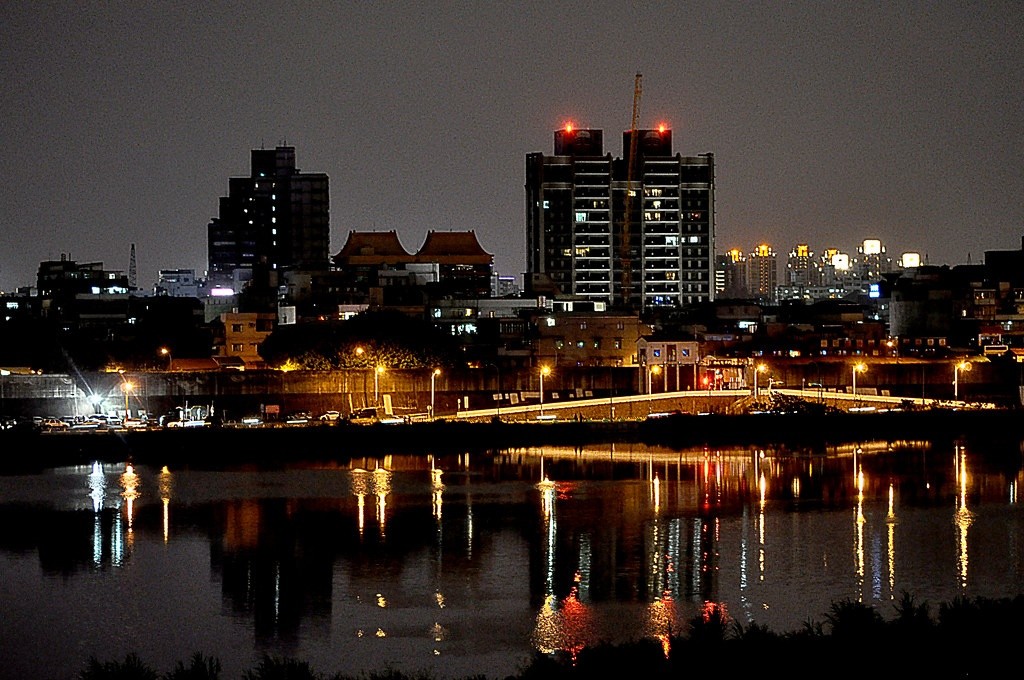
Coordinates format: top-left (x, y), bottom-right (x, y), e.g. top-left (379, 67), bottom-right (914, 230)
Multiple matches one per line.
top-left (352, 406), bottom-right (377, 419)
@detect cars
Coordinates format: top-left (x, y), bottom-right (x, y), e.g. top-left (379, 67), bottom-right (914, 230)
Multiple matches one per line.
top-left (318, 410), bottom-right (343, 422)
top-left (286, 412), bottom-right (313, 420)
top-left (32, 413), bottom-right (160, 430)
top-left (167, 418), bottom-right (192, 427)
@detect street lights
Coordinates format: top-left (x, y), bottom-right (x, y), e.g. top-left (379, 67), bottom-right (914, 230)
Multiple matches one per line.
top-left (954, 363), bottom-right (965, 400)
top-left (431, 369), bottom-right (442, 418)
top-left (540, 367), bottom-right (550, 417)
top-left (754, 365), bottom-right (764, 400)
top-left (648, 367), bottom-right (661, 414)
top-left (853, 364), bottom-right (863, 400)
top-left (161, 349), bottom-right (173, 371)
top-left (375, 364), bottom-right (385, 403)
top-left (125, 383), bottom-right (133, 415)
